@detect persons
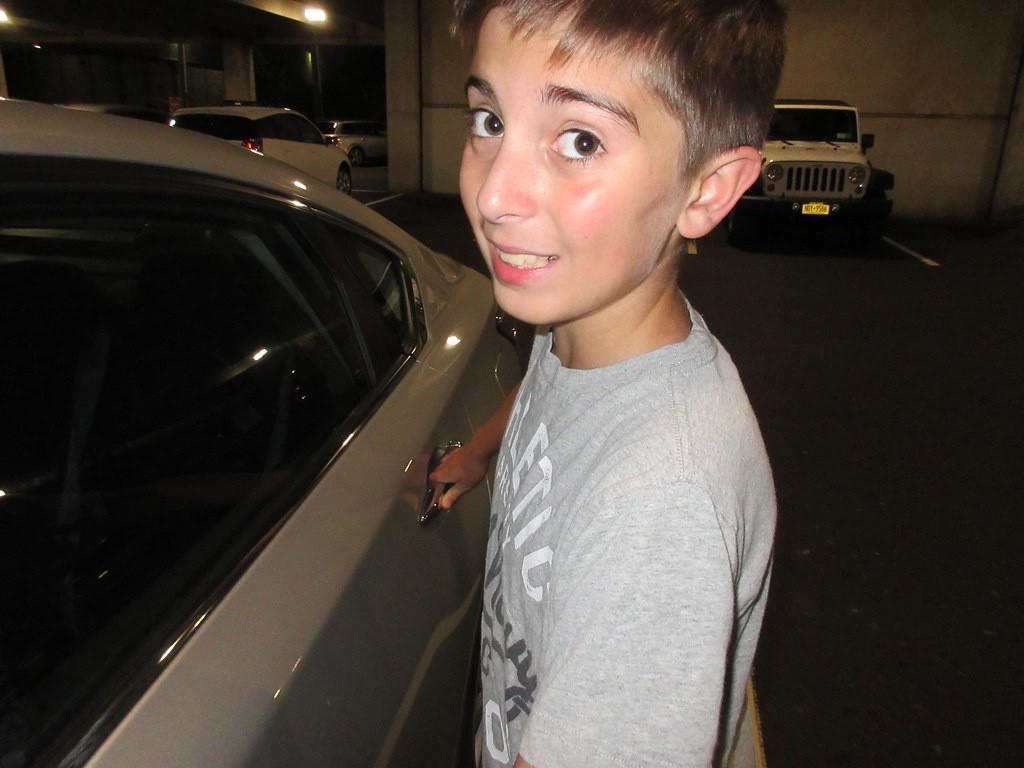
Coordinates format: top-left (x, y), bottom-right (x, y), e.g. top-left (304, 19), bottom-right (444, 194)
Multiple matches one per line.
top-left (422, 0), bottom-right (790, 768)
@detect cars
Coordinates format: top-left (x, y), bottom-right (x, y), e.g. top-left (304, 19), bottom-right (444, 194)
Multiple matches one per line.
top-left (0, 99), bottom-right (535, 768)
top-left (163, 104), bottom-right (351, 201)
top-left (312, 118), bottom-right (389, 168)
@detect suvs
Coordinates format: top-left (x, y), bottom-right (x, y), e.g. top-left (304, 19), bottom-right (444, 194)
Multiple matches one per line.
top-left (720, 96), bottom-right (877, 252)
